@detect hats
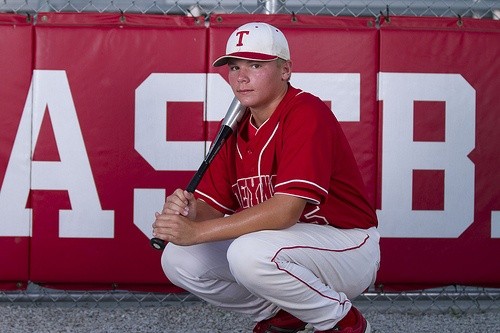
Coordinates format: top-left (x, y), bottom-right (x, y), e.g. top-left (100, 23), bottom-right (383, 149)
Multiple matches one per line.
top-left (212, 22), bottom-right (290, 67)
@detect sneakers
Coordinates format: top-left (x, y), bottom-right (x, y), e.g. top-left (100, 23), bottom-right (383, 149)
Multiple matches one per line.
top-left (252, 309), bottom-right (313, 333)
top-left (313, 306), bottom-right (371, 333)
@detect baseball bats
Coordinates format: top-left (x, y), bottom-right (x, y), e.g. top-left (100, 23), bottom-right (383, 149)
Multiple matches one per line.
top-left (150, 94), bottom-right (246, 250)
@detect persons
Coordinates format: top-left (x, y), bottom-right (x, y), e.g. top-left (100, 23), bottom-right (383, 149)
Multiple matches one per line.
top-left (151, 22), bottom-right (380, 333)
